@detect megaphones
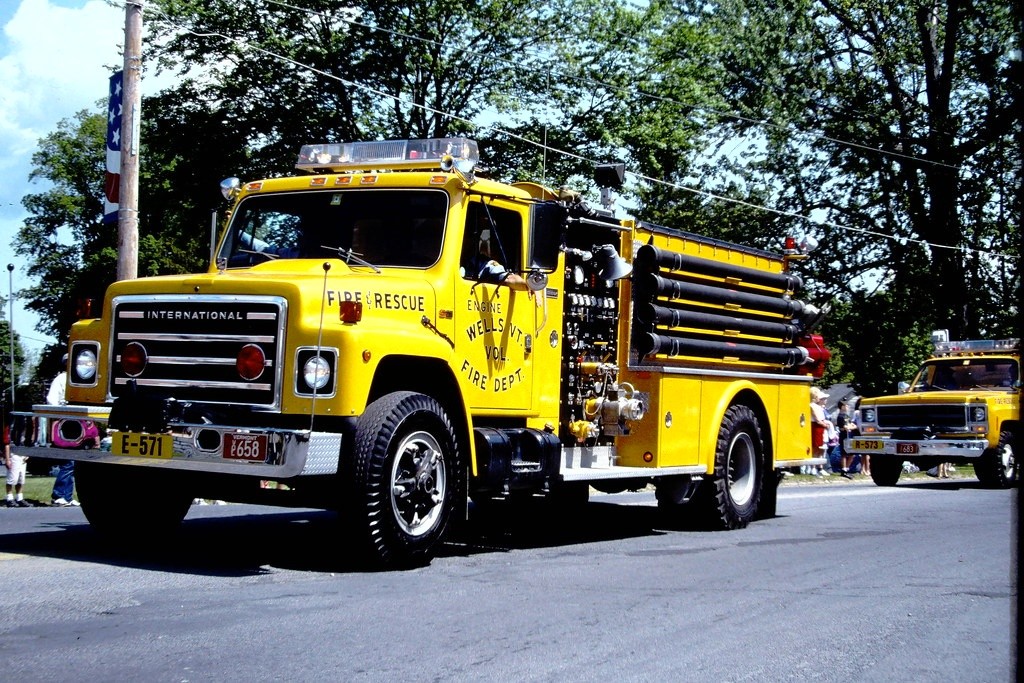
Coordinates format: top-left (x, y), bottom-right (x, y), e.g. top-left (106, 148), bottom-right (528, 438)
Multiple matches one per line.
top-left (598, 244), bottom-right (635, 281)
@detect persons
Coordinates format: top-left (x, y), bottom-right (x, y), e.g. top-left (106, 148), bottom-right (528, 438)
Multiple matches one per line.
top-left (224, 209), bottom-right (298, 258)
top-left (427, 226), bottom-right (530, 293)
top-left (800, 387), bottom-right (871, 477)
top-left (936, 463), bottom-right (948, 478)
top-left (2, 389), bottom-right (33, 507)
top-left (46, 354), bottom-right (80, 507)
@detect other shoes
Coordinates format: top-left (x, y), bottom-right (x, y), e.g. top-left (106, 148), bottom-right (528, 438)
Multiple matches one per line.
top-left (15, 499), bottom-right (34, 507)
top-left (70, 499), bottom-right (81, 507)
top-left (841, 470), bottom-right (853, 480)
top-left (818, 470), bottom-right (830, 477)
top-left (782, 471), bottom-right (794, 476)
top-left (51, 498), bottom-right (71, 507)
top-left (801, 465), bottom-right (811, 474)
top-left (811, 468), bottom-right (817, 476)
top-left (861, 470), bottom-right (871, 476)
top-left (6, 500), bottom-right (19, 508)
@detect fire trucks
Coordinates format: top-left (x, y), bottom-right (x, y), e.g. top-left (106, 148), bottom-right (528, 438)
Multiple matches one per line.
top-left (843, 330), bottom-right (1024, 490)
top-left (9, 136), bottom-right (828, 576)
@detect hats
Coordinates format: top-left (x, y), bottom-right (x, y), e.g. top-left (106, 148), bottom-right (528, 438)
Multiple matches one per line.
top-left (814, 392), bottom-right (830, 403)
